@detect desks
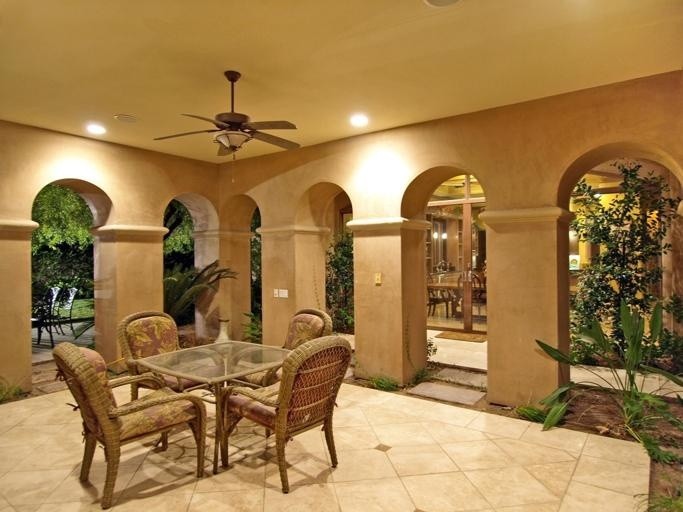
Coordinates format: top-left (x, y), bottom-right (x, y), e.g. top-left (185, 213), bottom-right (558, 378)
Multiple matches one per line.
top-left (428, 282), bottom-right (486, 318)
top-left (129, 340), bottom-right (293, 473)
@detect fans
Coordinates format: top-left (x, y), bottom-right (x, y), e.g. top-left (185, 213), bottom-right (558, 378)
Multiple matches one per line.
top-left (152, 71), bottom-right (300, 183)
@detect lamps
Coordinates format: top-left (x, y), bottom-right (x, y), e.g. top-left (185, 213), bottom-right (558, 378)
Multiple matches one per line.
top-left (216, 128), bottom-right (250, 183)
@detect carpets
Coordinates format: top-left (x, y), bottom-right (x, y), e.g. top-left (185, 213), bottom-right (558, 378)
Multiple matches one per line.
top-left (435, 329), bottom-right (487, 343)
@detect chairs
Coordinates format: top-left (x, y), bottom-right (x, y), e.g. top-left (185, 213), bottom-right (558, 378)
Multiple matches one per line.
top-left (458, 273), bottom-right (482, 315)
top-left (229, 309), bottom-right (333, 391)
top-left (51, 342), bottom-right (207, 510)
top-left (32, 286), bottom-right (79, 348)
top-left (219, 337), bottom-right (352, 492)
top-left (119, 311), bottom-right (223, 451)
top-left (425, 271), bottom-right (455, 319)
